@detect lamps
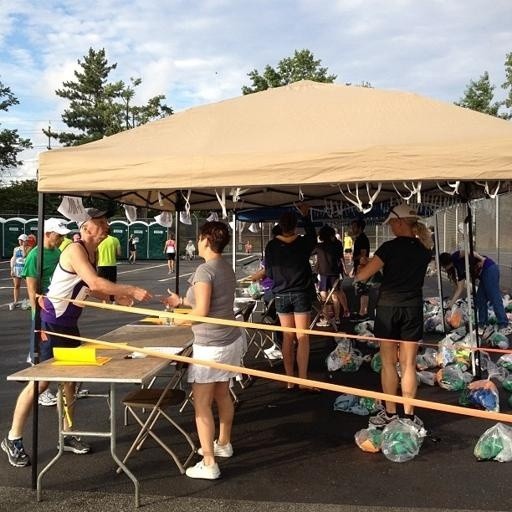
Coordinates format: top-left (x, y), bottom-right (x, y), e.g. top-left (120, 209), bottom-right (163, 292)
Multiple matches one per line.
top-left (245, 300), bottom-right (282, 368)
top-left (115, 346), bottom-right (198, 475)
top-left (308, 273), bottom-right (343, 332)
top-left (228, 312), bottom-right (252, 403)
top-left (243, 296), bottom-right (282, 358)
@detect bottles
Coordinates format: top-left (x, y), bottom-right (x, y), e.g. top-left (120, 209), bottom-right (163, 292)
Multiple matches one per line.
top-left (18, 234), bottom-right (27, 241)
top-left (44, 218), bottom-right (73, 235)
top-left (75, 207), bottom-right (107, 228)
top-left (382, 204), bottom-right (417, 226)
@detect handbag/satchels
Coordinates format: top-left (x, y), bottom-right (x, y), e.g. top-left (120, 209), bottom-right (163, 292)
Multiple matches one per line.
top-left (110, 295), bottom-right (115, 302)
top-left (179, 296), bottom-right (184, 305)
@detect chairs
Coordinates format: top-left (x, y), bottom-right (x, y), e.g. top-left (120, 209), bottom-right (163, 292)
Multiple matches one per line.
top-left (78, 389), bottom-right (89, 399)
top-left (38, 388), bottom-right (57, 406)
top-left (332, 318), bottom-right (340, 324)
top-left (185, 460), bottom-right (221, 480)
top-left (198, 440), bottom-right (233, 458)
top-left (1, 434), bottom-right (30, 468)
top-left (57, 434), bottom-right (90, 454)
top-left (405, 415), bottom-right (424, 427)
top-left (368, 409), bottom-right (400, 427)
top-left (264, 345), bottom-right (283, 360)
top-left (316, 319), bottom-right (331, 327)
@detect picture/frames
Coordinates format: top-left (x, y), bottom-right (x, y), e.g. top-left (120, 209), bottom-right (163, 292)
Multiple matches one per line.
top-left (124, 309), bottom-right (193, 325)
top-left (233, 275), bottom-right (272, 322)
top-left (6, 325), bottom-right (201, 508)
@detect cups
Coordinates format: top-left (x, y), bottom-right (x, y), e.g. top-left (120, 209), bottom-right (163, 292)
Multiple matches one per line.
top-left (388, 206), bottom-right (400, 219)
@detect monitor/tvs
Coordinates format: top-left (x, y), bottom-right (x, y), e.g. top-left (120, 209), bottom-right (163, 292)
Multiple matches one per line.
top-left (52, 346), bottom-right (112, 366)
top-left (144, 346), bottom-right (184, 355)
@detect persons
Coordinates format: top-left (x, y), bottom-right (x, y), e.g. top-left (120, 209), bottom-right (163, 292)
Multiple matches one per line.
top-left (245, 239), bottom-right (252, 255)
top-left (0, 207), bottom-right (154, 468)
top-left (355, 204), bottom-right (433, 427)
top-left (72, 233), bottom-right (81, 242)
top-left (439, 249), bottom-right (509, 336)
top-left (185, 239), bottom-right (196, 261)
top-left (348, 218), bottom-right (370, 320)
top-left (128, 233), bottom-right (137, 265)
top-left (312, 224), bottom-right (352, 327)
top-left (163, 232), bottom-right (175, 275)
top-left (93, 226), bottom-right (121, 309)
top-left (17, 216), bottom-right (89, 407)
top-left (7, 233), bottom-right (32, 311)
top-left (59, 233), bottom-right (72, 252)
top-left (252, 224), bottom-right (285, 360)
top-left (264, 200), bottom-right (321, 393)
top-left (427, 224), bottom-right (439, 260)
top-left (159, 221), bottom-right (244, 480)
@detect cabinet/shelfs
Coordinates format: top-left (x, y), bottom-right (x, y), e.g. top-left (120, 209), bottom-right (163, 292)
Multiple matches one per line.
top-left (164, 305), bottom-right (175, 327)
